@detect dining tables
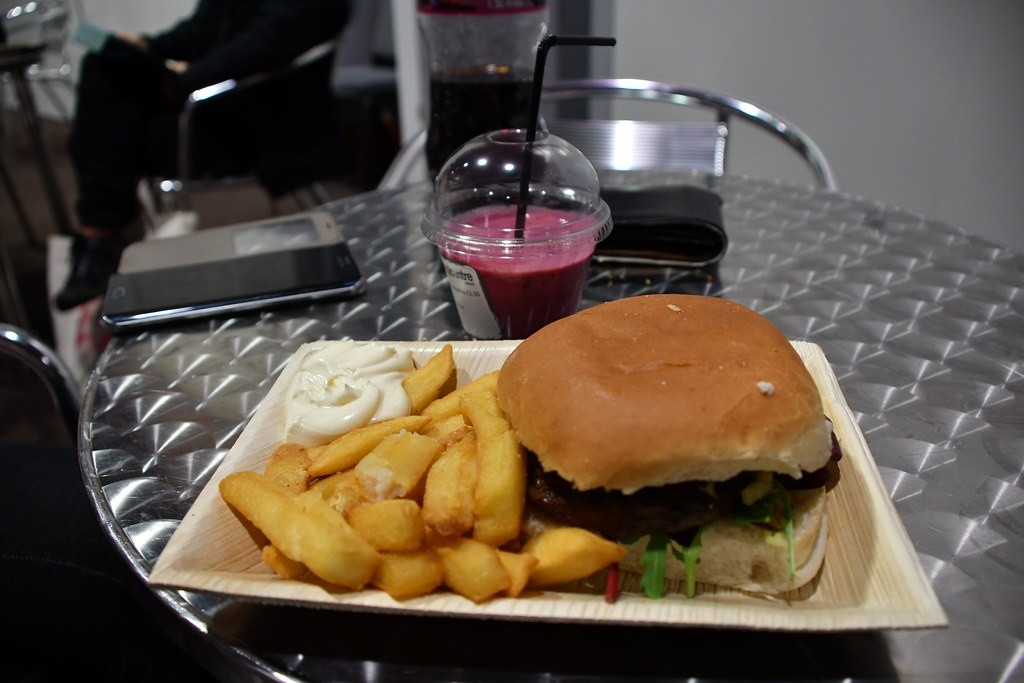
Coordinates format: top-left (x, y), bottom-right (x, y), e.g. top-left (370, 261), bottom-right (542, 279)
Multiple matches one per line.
top-left (80, 180), bottom-right (1024, 683)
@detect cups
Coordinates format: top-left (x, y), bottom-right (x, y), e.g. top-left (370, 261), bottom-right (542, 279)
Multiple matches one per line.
top-left (421, 130), bottom-right (614, 343)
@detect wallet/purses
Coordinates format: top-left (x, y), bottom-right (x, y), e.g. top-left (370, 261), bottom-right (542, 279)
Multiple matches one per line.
top-left (592, 184), bottom-right (729, 267)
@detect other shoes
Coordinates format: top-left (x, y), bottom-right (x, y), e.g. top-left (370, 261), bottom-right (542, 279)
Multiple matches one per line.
top-left (52, 221), bottom-right (147, 311)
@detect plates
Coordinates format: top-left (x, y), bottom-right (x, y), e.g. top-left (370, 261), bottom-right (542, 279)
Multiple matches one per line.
top-left (148, 336), bottom-right (949, 629)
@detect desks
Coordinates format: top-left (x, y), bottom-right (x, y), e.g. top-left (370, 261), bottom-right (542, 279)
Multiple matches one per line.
top-left (0, 0), bottom-right (71, 266)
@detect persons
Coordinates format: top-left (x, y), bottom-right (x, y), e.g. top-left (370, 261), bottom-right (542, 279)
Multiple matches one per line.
top-left (53, 0), bottom-right (353, 312)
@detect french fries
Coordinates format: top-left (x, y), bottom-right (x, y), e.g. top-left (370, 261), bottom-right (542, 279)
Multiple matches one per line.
top-left (218, 344), bottom-right (630, 605)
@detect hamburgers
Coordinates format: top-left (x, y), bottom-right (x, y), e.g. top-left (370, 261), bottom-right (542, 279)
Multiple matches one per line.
top-left (497, 294), bottom-right (843, 598)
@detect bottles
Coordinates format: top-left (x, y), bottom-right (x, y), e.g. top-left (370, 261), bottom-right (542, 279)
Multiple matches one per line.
top-left (418, 0), bottom-right (550, 187)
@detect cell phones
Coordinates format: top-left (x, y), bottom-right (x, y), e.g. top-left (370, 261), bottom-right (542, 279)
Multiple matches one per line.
top-left (116, 207), bottom-right (340, 274)
top-left (99, 240), bottom-right (367, 333)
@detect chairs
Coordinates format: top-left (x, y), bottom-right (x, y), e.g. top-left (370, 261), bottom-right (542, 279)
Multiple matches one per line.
top-left (163, 37), bottom-right (341, 197)
top-left (0, 321), bottom-right (186, 683)
top-left (382, 78), bottom-right (832, 192)
